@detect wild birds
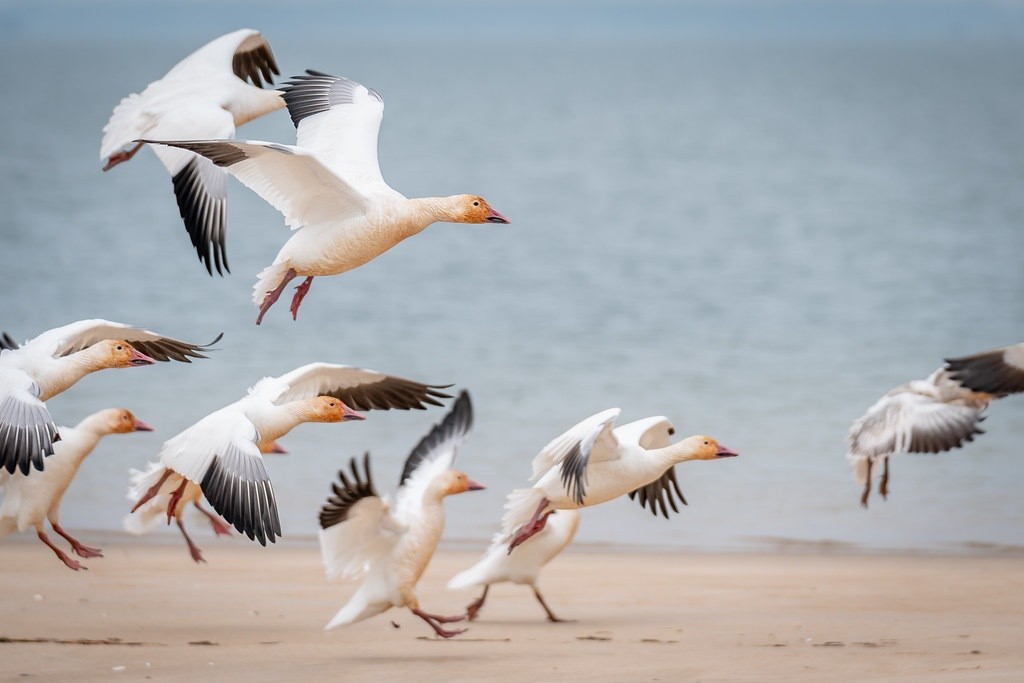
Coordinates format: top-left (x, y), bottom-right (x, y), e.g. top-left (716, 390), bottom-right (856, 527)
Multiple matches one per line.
top-left (505, 407), bottom-right (739, 556)
top-left (943, 342), bottom-right (1024, 399)
top-left (129, 361), bottom-right (457, 548)
top-left (120, 443), bottom-right (289, 565)
top-left (99, 28), bottom-right (289, 276)
top-left (0, 409), bottom-right (155, 572)
top-left (317, 388), bottom-right (486, 638)
top-left (131, 70), bottom-right (511, 324)
top-left (844, 361), bottom-right (1008, 510)
top-left (445, 486), bottom-right (580, 623)
top-left (0, 317), bottom-right (222, 473)
